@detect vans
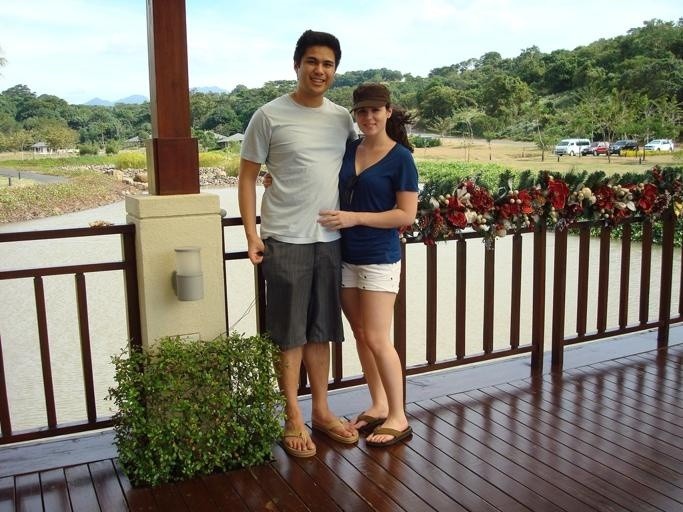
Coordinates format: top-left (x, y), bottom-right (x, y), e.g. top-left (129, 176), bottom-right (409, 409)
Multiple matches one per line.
top-left (554, 137), bottom-right (590, 156)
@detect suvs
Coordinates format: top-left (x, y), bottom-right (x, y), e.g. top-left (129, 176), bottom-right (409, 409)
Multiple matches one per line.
top-left (580, 141), bottom-right (610, 156)
top-left (606, 139), bottom-right (638, 155)
top-left (642, 138), bottom-right (674, 153)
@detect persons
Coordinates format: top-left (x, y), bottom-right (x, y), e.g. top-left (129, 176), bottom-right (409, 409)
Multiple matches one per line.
top-left (261, 82), bottom-right (418, 448)
top-left (236, 28), bottom-right (358, 460)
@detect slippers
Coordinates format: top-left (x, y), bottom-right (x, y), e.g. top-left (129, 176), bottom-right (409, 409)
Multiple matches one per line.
top-left (353, 409), bottom-right (387, 432)
top-left (312, 416), bottom-right (360, 444)
top-left (366, 424), bottom-right (413, 447)
top-left (279, 430), bottom-right (318, 458)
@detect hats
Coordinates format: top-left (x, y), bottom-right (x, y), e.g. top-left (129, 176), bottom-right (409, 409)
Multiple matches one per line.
top-left (349, 82), bottom-right (390, 113)
